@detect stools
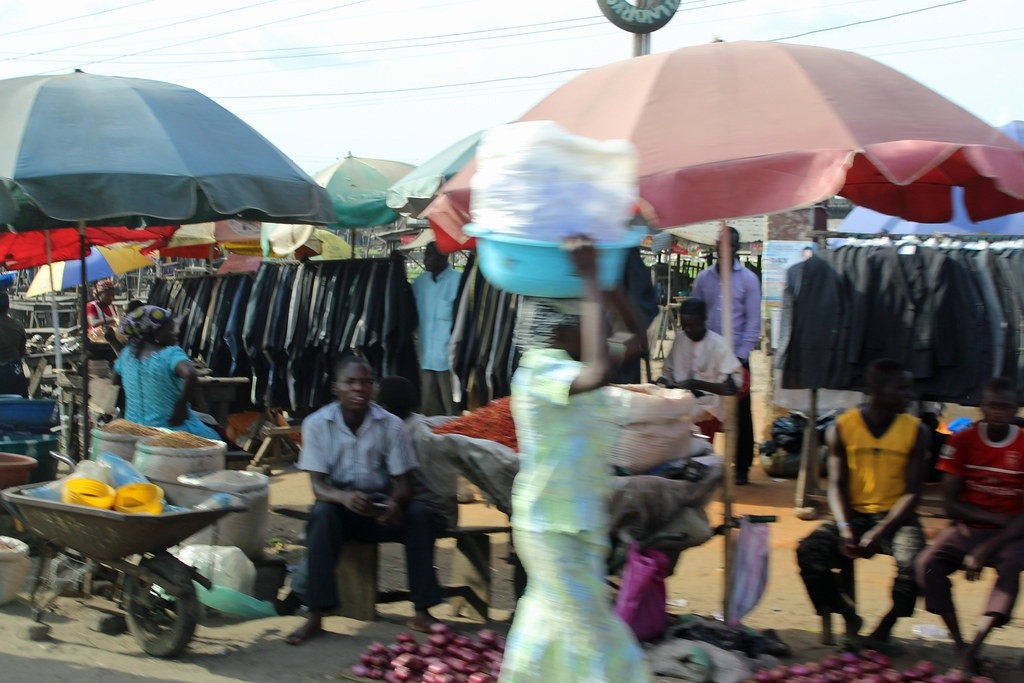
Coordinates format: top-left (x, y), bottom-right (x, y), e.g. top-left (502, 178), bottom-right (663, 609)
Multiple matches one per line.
top-left (254, 426), bottom-right (302, 463)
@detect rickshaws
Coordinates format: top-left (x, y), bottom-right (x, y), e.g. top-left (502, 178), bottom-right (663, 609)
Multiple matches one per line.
top-left (0, 448), bottom-right (250, 659)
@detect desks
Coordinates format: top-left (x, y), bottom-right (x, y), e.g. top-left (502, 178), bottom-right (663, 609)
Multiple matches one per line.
top-left (26, 346), bottom-right (116, 396)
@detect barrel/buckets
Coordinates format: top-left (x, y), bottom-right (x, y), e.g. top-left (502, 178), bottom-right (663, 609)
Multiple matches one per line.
top-left (61, 478), bottom-right (114, 510)
top-left (113, 483), bottom-right (164, 513)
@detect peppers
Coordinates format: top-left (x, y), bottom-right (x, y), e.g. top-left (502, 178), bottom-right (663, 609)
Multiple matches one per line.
top-left (432, 396), bottom-right (516, 451)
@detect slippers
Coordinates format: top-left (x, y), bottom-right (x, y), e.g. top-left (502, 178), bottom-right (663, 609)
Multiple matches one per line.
top-left (283, 626), bottom-right (323, 646)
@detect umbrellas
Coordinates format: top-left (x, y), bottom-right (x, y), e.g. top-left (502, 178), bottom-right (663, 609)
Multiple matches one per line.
top-left (0, 40), bottom-right (1024, 625)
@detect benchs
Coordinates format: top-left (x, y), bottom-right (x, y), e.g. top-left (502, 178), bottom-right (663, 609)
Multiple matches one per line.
top-left (822, 543), bottom-right (934, 646)
top-left (273, 507), bottom-right (512, 622)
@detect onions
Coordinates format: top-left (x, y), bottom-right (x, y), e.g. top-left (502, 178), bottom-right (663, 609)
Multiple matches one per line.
top-left (351, 623), bottom-right (508, 683)
top-left (736, 646), bottom-right (996, 683)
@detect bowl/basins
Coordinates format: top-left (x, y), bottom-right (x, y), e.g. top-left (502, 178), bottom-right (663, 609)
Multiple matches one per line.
top-left (1, 399), bottom-right (55, 425)
top-left (458, 220), bottom-right (647, 297)
top-left (0, 452), bottom-right (38, 490)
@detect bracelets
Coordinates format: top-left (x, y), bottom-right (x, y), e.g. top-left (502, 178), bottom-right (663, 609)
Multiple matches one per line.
top-left (837, 522), bottom-right (851, 528)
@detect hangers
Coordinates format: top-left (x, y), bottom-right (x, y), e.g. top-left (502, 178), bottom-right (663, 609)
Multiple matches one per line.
top-left (846, 234), bottom-right (1024, 260)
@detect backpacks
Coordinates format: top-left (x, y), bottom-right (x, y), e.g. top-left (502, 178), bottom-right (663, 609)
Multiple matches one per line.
top-left (773, 412), bottom-right (836, 454)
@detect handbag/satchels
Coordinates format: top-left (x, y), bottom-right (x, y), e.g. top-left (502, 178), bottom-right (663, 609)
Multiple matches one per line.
top-left (616, 544), bottom-right (671, 645)
top-left (727, 519), bottom-right (771, 627)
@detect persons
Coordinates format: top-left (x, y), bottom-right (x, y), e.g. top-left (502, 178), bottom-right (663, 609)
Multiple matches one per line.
top-left (113, 305), bottom-right (222, 442)
top-left (0, 292), bottom-right (30, 400)
top-left (915, 378), bottom-right (1024, 675)
top-left (85, 280), bottom-right (118, 331)
top-left (412, 240), bottom-right (462, 416)
top-left (286, 355), bottom-right (445, 646)
top-left (687, 226), bottom-right (762, 485)
top-left (658, 298), bottom-right (744, 422)
top-left (497, 233), bottom-right (650, 683)
top-left (797, 360), bottom-right (931, 650)
